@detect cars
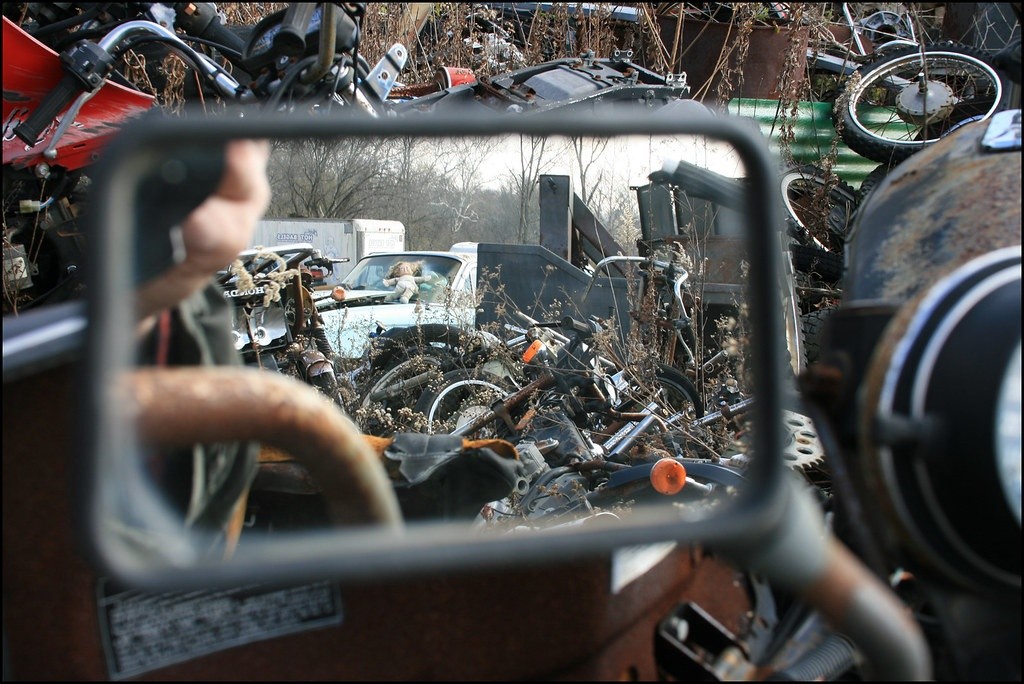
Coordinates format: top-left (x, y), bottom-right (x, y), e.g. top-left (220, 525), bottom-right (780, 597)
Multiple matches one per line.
top-left (317, 252), bottom-right (478, 356)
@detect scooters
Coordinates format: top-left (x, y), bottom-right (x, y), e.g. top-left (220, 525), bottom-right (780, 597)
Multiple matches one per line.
top-left (0, 0), bottom-right (1008, 530)
top-left (207, 241), bottom-right (749, 488)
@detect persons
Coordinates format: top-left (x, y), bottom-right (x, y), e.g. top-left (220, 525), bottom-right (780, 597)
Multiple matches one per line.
top-left (112, 141), bottom-right (271, 566)
top-left (384, 262), bottom-right (431, 304)
